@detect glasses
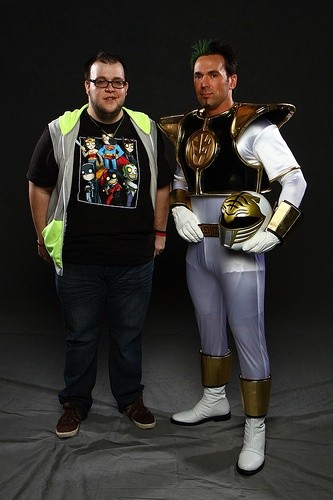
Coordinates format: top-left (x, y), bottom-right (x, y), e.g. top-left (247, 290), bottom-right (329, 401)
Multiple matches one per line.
top-left (88, 77), bottom-right (126, 89)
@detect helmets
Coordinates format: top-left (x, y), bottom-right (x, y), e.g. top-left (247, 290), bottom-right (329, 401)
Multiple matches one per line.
top-left (218, 191), bottom-right (273, 250)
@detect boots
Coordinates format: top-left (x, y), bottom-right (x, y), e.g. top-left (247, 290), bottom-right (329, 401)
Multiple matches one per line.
top-left (236, 416), bottom-right (266, 476)
top-left (171, 385), bottom-right (231, 426)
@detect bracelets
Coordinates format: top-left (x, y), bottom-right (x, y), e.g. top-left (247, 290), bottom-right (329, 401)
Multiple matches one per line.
top-left (37, 240), bottom-right (44, 246)
top-left (155, 230), bottom-right (167, 233)
top-left (155, 232), bottom-right (166, 236)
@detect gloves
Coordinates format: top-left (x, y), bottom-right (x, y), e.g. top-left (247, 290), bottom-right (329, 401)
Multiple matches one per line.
top-left (171, 205), bottom-right (204, 243)
top-left (243, 231), bottom-right (280, 254)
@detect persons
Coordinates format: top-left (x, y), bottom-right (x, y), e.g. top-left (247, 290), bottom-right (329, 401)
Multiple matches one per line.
top-left (27, 51), bottom-right (174, 438)
top-left (159, 40), bottom-right (308, 475)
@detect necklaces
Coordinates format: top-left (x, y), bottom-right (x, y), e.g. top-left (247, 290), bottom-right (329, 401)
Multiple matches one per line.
top-left (89, 114), bottom-right (125, 140)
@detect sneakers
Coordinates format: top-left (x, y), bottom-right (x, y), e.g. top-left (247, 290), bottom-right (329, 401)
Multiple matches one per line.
top-left (56, 408), bottom-right (81, 438)
top-left (119, 401), bottom-right (156, 428)
